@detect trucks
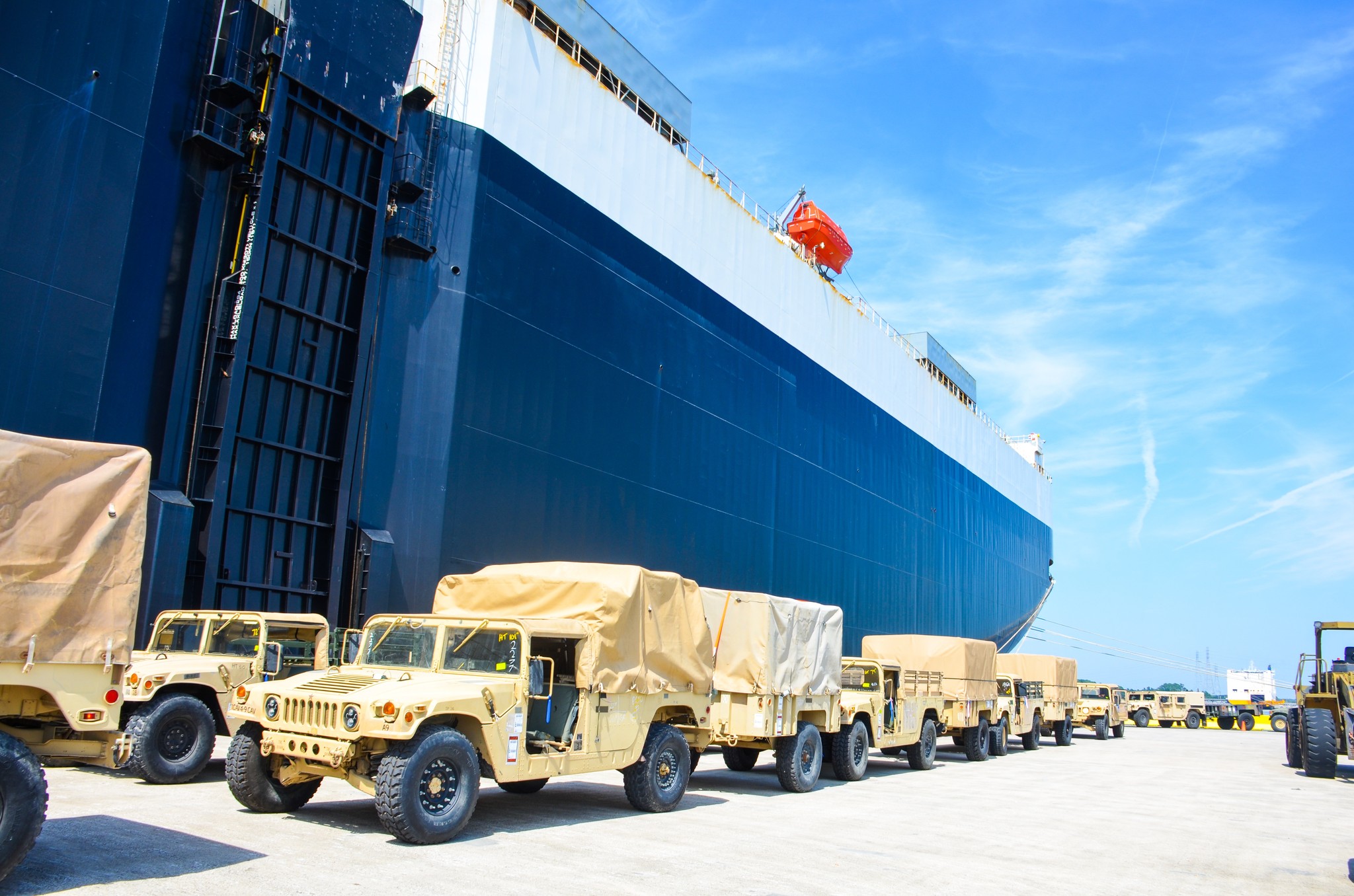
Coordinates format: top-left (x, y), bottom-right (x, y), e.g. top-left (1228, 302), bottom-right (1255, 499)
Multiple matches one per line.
top-left (821, 634), bottom-right (997, 781)
top-left (119, 610), bottom-right (365, 786)
top-left (226, 562), bottom-right (844, 846)
top-left (1128, 688), bottom-right (1297, 732)
top-left (952, 653), bottom-right (1079, 756)
top-left (1070, 683), bottom-right (1127, 740)
top-left (0, 430), bottom-right (154, 885)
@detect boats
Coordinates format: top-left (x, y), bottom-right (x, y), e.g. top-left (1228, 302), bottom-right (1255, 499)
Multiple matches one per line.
top-left (786, 200), bottom-right (853, 276)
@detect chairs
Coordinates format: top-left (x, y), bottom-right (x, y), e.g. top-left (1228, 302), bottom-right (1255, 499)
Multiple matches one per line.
top-left (528, 679), bottom-right (578, 748)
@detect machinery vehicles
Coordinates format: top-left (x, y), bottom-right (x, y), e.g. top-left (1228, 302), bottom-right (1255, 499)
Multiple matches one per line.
top-left (1285, 621), bottom-right (1354, 778)
